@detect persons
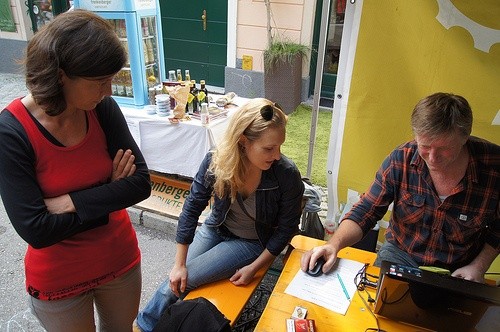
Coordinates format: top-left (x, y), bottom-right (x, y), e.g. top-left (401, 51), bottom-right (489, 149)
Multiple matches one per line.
top-left (0, 8), bottom-right (151, 332)
top-left (301, 92), bottom-right (500, 284)
top-left (132, 98), bottom-right (305, 332)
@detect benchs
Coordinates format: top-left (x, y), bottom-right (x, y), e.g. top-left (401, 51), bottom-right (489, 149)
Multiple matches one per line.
top-left (290, 234), bottom-right (378, 266)
top-left (172, 255), bottom-right (275, 329)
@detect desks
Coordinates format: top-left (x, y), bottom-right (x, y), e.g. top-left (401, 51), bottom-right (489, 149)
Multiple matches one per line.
top-left (252, 248), bottom-right (425, 332)
top-left (121, 91), bottom-right (243, 209)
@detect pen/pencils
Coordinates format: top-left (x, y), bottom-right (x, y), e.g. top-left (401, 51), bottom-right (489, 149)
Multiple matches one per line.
top-left (337, 273), bottom-right (352, 303)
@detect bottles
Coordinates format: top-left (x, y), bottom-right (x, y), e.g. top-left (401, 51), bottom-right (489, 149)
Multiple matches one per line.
top-left (201, 103), bottom-right (210, 127)
top-left (185, 70), bottom-right (190, 86)
top-left (106, 17), bottom-right (166, 107)
top-left (190, 80), bottom-right (198, 112)
top-left (177, 69), bottom-right (183, 87)
top-left (168, 70), bottom-right (177, 109)
top-left (198, 80), bottom-right (208, 112)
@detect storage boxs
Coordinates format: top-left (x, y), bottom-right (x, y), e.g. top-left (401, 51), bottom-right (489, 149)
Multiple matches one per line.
top-left (73, 1), bottom-right (167, 109)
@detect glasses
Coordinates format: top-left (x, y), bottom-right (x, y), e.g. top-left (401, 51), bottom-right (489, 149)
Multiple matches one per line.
top-left (260, 103), bottom-right (284, 118)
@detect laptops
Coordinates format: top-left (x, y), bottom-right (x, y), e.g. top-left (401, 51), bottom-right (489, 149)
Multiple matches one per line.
top-left (373, 260), bottom-right (500, 332)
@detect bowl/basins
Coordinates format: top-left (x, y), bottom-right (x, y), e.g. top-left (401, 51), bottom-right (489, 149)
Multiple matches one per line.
top-left (155, 94), bottom-right (171, 116)
top-left (144, 105), bottom-right (157, 114)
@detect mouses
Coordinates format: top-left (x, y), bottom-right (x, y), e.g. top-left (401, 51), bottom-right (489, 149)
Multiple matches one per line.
top-left (306, 258), bottom-right (326, 277)
